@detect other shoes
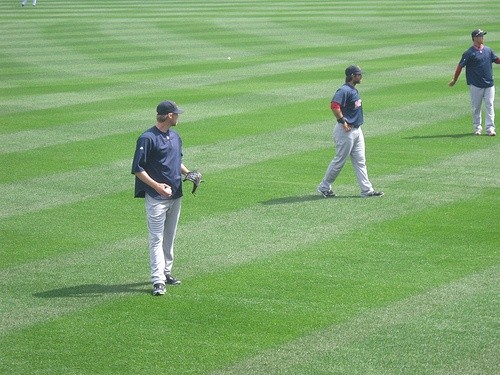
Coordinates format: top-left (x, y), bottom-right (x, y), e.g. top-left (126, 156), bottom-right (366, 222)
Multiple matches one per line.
top-left (364, 191), bottom-right (384, 198)
top-left (317, 186), bottom-right (336, 197)
top-left (474, 129), bottom-right (482, 135)
top-left (487, 129), bottom-right (496, 136)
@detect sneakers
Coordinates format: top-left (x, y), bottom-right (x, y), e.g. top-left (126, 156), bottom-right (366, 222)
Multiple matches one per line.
top-left (152, 284), bottom-right (167, 295)
top-left (165, 275), bottom-right (182, 286)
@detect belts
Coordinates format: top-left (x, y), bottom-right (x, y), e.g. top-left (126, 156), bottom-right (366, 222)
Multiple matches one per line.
top-left (350, 125), bottom-right (361, 128)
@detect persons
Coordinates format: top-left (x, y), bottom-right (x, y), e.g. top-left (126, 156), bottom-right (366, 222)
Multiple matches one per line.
top-left (317, 64), bottom-right (385, 199)
top-left (448, 29), bottom-right (500, 136)
top-left (131, 101), bottom-right (203, 296)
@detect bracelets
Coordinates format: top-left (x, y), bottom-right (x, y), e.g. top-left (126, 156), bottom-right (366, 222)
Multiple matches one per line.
top-left (337, 117), bottom-right (345, 124)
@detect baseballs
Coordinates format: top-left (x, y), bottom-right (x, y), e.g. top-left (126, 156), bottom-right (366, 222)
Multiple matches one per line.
top-left (165, 188), bottom-right (172, 195)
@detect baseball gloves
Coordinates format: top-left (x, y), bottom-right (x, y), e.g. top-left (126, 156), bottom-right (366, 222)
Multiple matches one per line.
top-left (183, 170), bottom-right (202, 194)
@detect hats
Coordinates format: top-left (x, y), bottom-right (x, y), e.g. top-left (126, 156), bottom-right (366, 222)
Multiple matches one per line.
top-left (345, 65), bottom-right (366, 76)
top-left (156, 100), bottom-right (184, 115)
top-left (471, 29), bottom-right (488, 38)
